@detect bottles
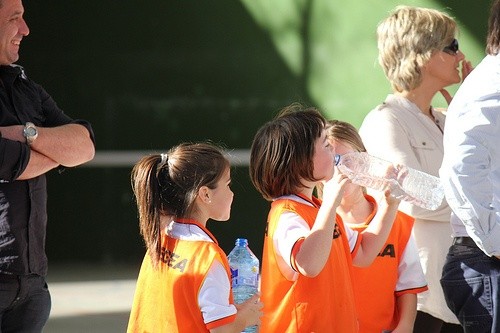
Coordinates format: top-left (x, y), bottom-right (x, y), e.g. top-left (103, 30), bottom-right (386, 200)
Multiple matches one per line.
top-left (333, 152), bottom-right (444, 211)
top-left (226, 238), bottom-right (260, 333)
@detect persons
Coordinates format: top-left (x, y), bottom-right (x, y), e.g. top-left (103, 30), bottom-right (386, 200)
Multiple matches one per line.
top-left (327, 120), bottom-right (428, 333)
top-left (0, 0), bottom-right (96, 333)
top-left (125, 141), bottom-right (264, 333)
top-left (250, 102), bottom-right (405, 333)
top-left (359, 6), bottom-right (474, 333)
top-left (439, 0), bottom-right (500, 333)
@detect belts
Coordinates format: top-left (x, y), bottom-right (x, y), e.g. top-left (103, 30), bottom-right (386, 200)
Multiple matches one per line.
top-left (453, 236), bottom-right (478, 247)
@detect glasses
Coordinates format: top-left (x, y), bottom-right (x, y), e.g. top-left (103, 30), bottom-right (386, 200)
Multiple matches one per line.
top-left (443, 39), bottom-right (459, 55)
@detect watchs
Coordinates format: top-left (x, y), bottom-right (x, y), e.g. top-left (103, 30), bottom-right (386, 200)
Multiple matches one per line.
top-left (24, 122), bottom-right (38, 146)
